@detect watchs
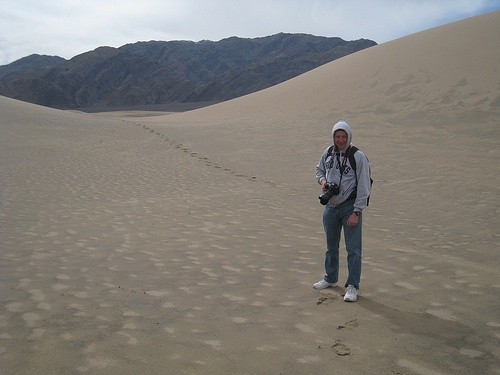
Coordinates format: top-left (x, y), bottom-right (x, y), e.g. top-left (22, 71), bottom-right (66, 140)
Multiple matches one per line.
top-left (354, 211), bottom-right (361, 216)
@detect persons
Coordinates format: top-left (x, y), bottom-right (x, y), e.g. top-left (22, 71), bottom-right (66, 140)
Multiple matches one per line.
top-left (313, 120), bottom-right (373, 302)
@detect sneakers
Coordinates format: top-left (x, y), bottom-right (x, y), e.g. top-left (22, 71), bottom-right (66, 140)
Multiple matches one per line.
top-left (313, 279), bottom-right (338, 290)
top-left (344, 284), bottom-right (360, 301)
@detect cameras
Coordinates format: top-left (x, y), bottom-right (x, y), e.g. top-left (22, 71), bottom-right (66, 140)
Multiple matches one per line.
top-left (318, 182), bottom-right (339, 205)
